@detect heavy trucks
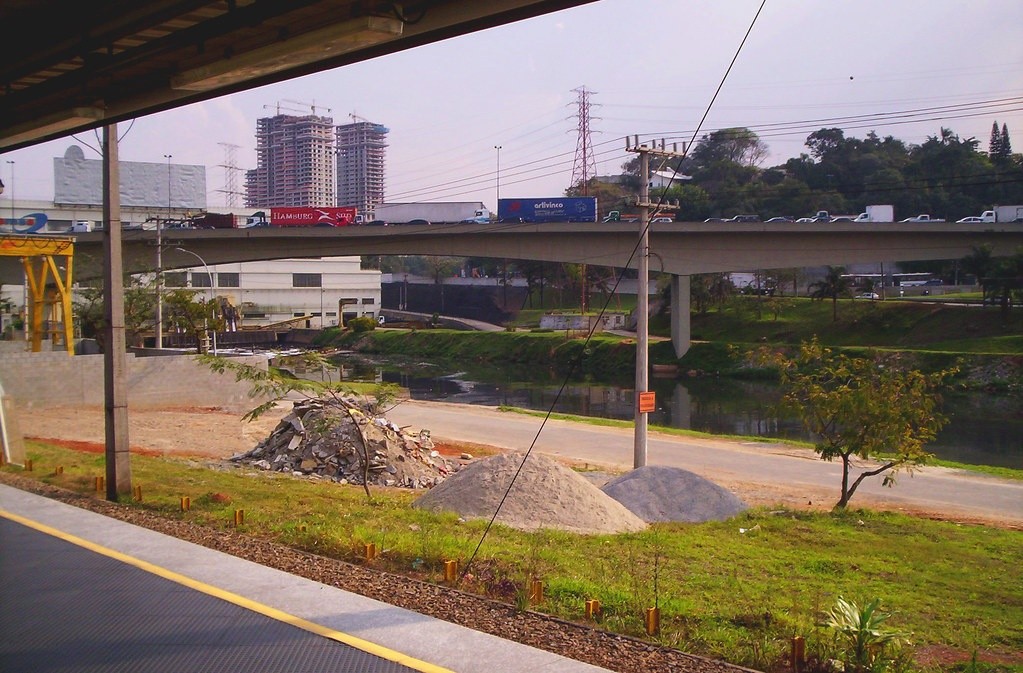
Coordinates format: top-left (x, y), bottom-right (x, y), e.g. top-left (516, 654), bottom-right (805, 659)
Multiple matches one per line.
top-left (468, 195), bottom-right (599, 224)
top-left (602, 211), bottom-right (677, 224)
top-left (356, 201), bottom-right (488, 224)
top-left (244, 206), bottom-right (357, 228)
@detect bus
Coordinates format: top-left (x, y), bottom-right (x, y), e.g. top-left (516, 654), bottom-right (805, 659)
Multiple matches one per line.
top-left (839, 272), bottom-right (936, 289)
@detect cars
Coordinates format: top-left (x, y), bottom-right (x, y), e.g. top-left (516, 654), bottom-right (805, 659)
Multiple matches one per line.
top-left (739, 284), bottom-right (775, 297)
top-left (828, 217), bottom-right (853, 223)
top-left (984, 295), bottom-right (1012, 306)
top-left (69, 195), bottom-right (493, 233)
top-left (897, 217), bottom-right (917, 223)
top-left (815, 210), bottom-right (859, 220)
top-left (854, 293), bottom-right (880, 300)
top-left (920, 278), bottom-right (943, 287)
top-left (955, 216), bottom-right (983, 224)
top-left (628, 210), bottom-right (818, 224)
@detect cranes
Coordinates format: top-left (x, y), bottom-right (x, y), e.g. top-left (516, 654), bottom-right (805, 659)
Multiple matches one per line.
top-left (288, 98), bottom-right (333, 116)
top-left (343, 108), bottom-right (370, 124)
top-left (263, 101), bottom-right (307, 117)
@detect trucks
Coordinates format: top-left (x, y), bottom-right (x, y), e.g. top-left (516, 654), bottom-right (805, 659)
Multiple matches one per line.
top-left (980, 204), bottom-right (1023, 224)
top-left (853, 205), bottom-right (894, 223)
top-left (191, 211), bottom-right (238, 230)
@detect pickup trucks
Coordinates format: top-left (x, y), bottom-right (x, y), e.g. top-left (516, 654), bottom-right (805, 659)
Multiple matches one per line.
top-left (915, 214), bottom-right (946, 223)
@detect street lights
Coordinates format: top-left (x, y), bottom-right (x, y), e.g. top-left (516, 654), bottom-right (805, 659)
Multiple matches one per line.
top-left (174, 247), bottom-right (217, 358)
top-left (494, 145), bottom-right (502, 198)
top-left (162, 155), bottom-right (172, 223)
top-left (6, 160), bottom-right (14, 233)
